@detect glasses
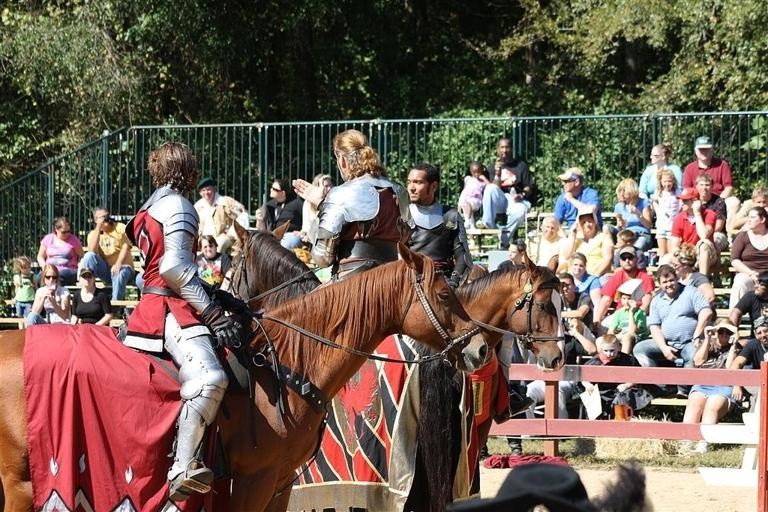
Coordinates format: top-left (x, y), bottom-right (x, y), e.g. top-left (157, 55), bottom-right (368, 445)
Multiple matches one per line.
top-left (55, 227), bottom-right (73, 235)
top-left (268, 182), bottom-right (284, 195)
top-left (43, 273), bottom-right (58, 281)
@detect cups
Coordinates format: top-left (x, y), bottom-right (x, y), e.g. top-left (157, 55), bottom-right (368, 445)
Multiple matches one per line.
top-left (614, 403), bottom-right (633, 421)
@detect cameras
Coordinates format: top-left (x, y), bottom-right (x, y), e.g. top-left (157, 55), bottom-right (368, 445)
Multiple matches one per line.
top-left (103, 213), bottom-right (114, 225)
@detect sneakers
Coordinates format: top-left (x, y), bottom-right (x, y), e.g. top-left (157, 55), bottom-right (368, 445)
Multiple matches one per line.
top-left (677, 440), bottom-right (711, 458)
top-left (165, 465), bottom-right (216, 501)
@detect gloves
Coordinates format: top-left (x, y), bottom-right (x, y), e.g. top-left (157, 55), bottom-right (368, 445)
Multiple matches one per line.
top-left (199, 301), bottom-right (244, 350)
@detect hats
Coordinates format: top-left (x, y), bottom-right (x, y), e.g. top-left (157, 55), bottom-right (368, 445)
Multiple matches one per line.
top-left (557, 165), bottom-right (585, 185)
top-left (619, 243), bottom-right (638, 258)
top-left (196, 177), bottom-right (217, 191)
top-left (79, 267), bottom-right (96, 279)
top-left (693, 133), bottom-right (716, 153)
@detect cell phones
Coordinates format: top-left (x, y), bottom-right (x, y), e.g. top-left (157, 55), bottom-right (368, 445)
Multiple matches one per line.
top-left (500, 154), bottom-right (505, 163)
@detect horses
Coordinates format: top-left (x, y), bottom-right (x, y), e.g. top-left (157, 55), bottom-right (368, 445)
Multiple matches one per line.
top-left (230, 219), bottom-right (463, 512)
top-left (0, 242), bottom-right (489, 511)
top-left (455, 248), bottom-right (565, 372)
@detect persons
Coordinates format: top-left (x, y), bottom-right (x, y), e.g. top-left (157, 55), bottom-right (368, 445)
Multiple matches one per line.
top-left (406, 163), bottom-right (473, 291)
top-left (123, 140), bottom-right (245, 502)
top-left (12, 172), bottom-right (334, 328)
top-left (458, 136), bottom-right (768, 459)
top-left (291, 129), bottom-right (416, 282)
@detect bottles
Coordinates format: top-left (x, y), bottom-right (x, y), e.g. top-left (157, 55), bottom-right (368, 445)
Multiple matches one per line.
top-left (509, 186), bottom-right (517, 198)
